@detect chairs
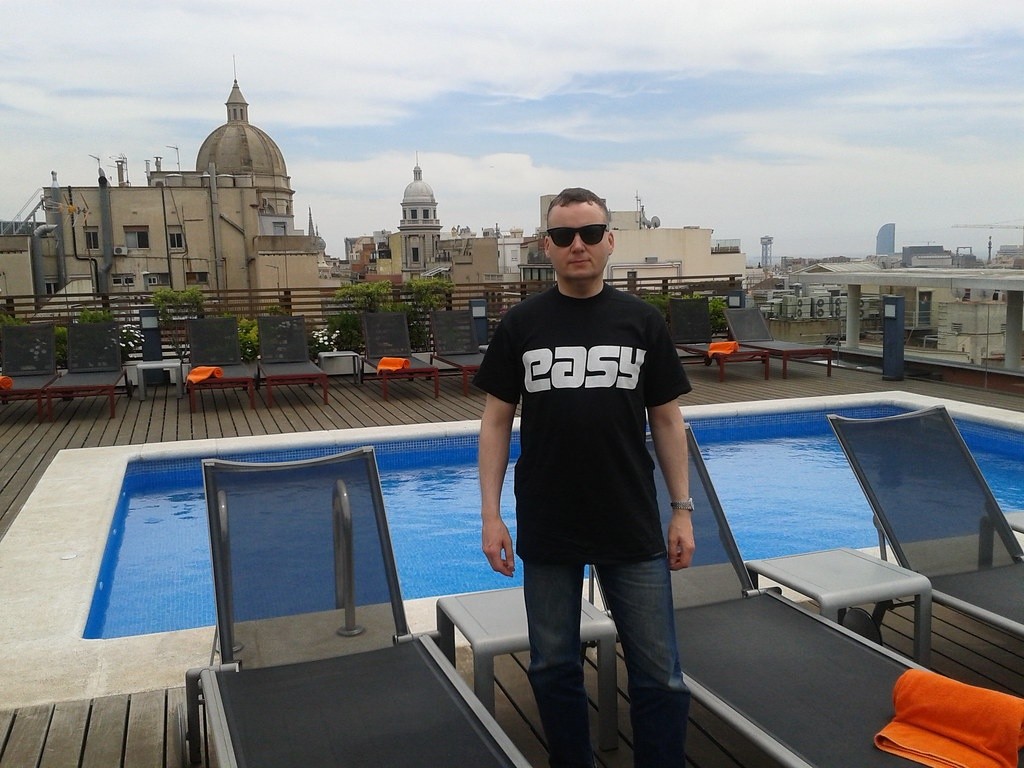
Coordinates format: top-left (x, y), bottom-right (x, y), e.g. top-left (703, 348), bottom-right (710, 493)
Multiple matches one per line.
top-left (581, 421), bottom-right (1024, 768)
top-left (0, 321), bottom-right (134, 424)
top-left (183, 315), bottom-right (328, 413)
top-left (666, 298), bottom-right (833, 381)
top-left (185, 446), bottom-right (536, 768)
top-left (826, 403), bottom-right (1024, 639)
top-left (361, 309), bottom-right (486, 400)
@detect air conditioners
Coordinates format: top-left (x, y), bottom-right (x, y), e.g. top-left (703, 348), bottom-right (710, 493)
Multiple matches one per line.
top-left (782, 295), bottom-right (870, 320)
top-left (113, 246), bottom-right (127, 256)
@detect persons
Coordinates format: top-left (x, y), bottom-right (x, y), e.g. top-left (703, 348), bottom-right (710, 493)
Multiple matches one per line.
top-left (471, 187), bottom-right (695, 768)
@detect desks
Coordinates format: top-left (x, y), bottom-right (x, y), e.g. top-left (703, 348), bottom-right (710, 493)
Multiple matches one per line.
top-left (745, 547), bottom-right (933, 670)
top-left (317, 351), bottom-right (361, 390)
top-left (436, 586), bottom-right (619, 751)
top-left (137, 359), bottom-right (183, 401)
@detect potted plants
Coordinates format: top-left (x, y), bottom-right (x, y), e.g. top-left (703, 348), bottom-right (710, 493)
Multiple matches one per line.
top-left (149, 276), bottom-right (455, 379)
top-left (643, 291), bottom-right (729, 357)
top-left (1, 303), bottom-right (141, 384)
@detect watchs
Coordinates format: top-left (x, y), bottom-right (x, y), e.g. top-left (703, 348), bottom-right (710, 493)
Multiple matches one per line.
top-left (671, 497), bottom-right (695, 512)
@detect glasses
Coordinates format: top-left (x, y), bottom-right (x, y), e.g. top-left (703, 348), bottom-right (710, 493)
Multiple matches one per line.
top-left (547, 223), bottom-right (609, 247)
